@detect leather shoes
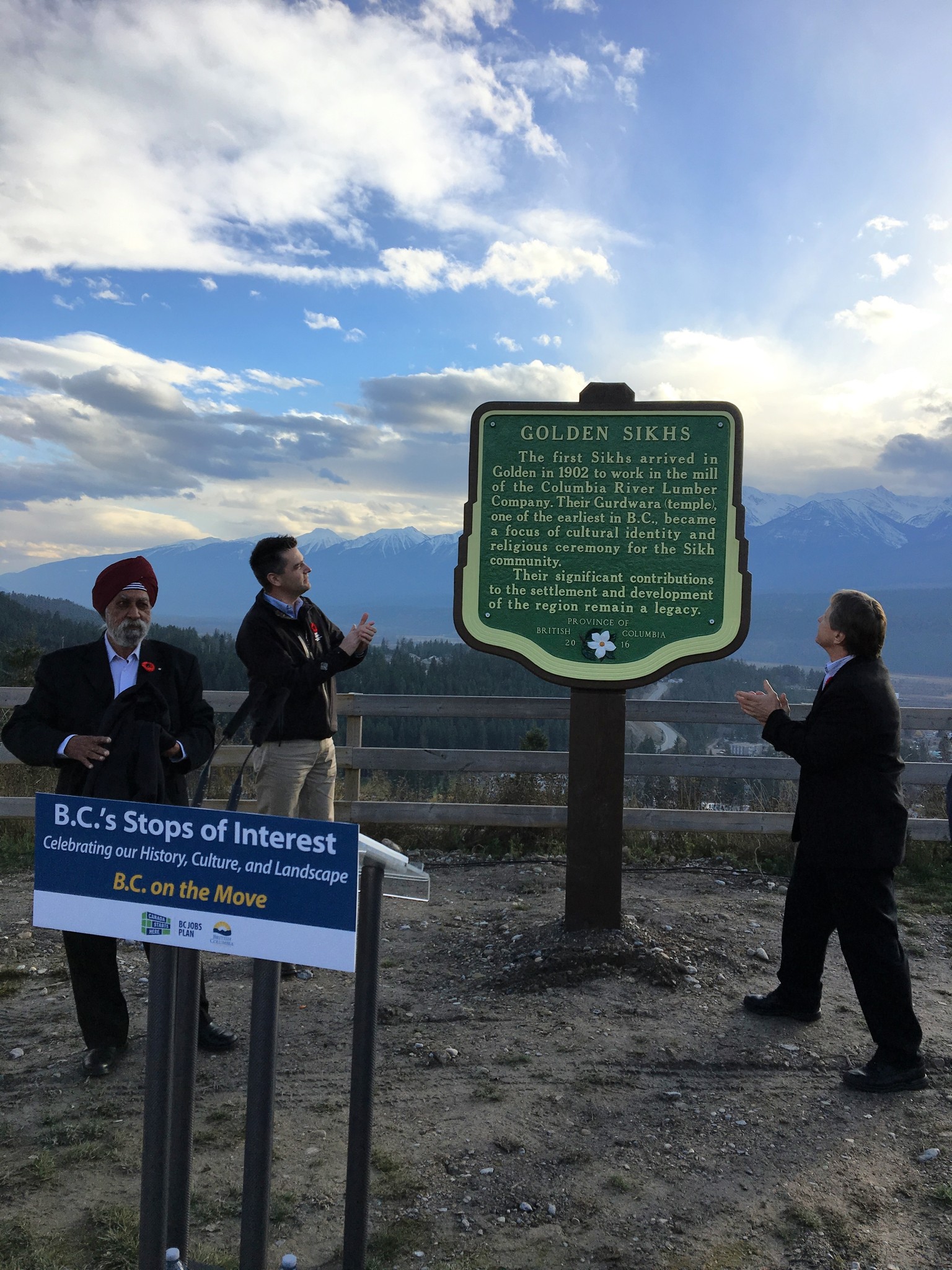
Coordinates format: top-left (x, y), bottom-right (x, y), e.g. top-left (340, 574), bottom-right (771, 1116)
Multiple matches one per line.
top-left (842, 1057), bottom-right (928, 1090)
top-left (196, 1021), bottom-right (240, 1050)
top-left (80, 1039), bottom-right (129, 1076)
top-left (743, 990), bottom-right (822, 1020)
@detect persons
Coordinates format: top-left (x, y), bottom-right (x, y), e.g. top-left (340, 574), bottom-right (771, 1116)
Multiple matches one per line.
top-left (236, 534), bottom-right (376, 975)
top-left (734, 588), bottom-right (929, 1093)
top-left (1, 555), bottom-right (236, 1076)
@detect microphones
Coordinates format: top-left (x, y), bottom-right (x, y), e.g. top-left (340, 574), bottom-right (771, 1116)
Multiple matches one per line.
top-left (190, 684), bottom-right (264, 808)
top-left (224, 686), bottom-right (290, 811)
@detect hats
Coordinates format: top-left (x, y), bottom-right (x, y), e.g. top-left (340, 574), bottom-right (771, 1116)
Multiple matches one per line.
top-left (92, 556), bottom-right (159, 614)
